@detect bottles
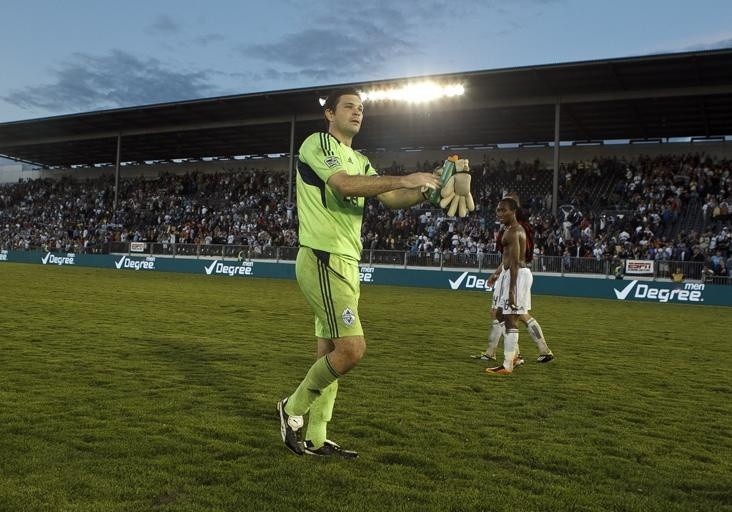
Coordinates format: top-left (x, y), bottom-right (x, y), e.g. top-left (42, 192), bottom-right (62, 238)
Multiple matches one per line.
top-left (425, 154), bottom-right (458, 206)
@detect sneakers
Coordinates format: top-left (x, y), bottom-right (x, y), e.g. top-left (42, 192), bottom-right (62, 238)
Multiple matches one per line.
top-left (486, 366), bottom-right (513, 375)
top-left (303, 439), bottom-right (359, 459)
top-left (513, 355), bottom-right (524, 368)
top-left (276, 397), bottom-right (306, 456)
top-left (538, 350), bottom-right (554, 362)
top-left (470, 352), bottom-right (497, 361)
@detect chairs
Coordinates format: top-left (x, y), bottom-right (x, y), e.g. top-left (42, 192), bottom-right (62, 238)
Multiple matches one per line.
top-left (474, 158), bottom-right (707, 260)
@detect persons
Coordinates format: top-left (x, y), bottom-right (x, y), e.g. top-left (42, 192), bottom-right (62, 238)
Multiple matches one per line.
top-left (485, 198), bottom-right (526, 376)
top-left (361, 153), bottom-right (732, 283)
top-left (1, 166), bottom-right (299, 262)
top-left (469, 191), bottom-right (554, 364)
top-left (275, 87), bottom-right (445, 461)
